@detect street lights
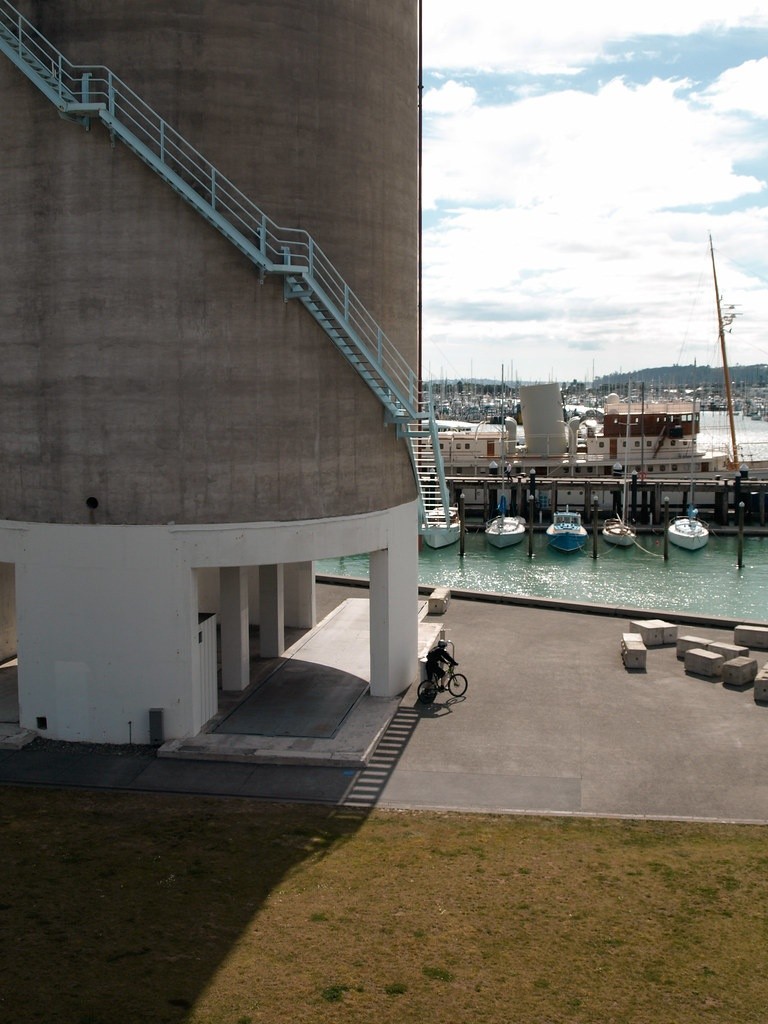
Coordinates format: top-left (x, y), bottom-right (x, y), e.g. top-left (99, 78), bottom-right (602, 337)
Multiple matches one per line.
top-left (556, 419), bottom-right (575, 479)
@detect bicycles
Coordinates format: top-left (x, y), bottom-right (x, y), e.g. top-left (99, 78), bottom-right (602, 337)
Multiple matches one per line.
top-left (417, 661), bottom-right (468, 704)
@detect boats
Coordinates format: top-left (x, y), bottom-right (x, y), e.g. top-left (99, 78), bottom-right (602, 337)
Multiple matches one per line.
top-left (545, 503), bottom-right (588, 551)
top-left (420, 505), bottom-right (460, 549)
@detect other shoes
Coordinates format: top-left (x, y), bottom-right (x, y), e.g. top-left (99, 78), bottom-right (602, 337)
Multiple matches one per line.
top-left (441, 685), bottom-right (448, 690)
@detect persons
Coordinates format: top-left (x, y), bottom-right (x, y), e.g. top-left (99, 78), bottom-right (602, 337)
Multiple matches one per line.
top-left (424, 639), bottom-right (458, 693)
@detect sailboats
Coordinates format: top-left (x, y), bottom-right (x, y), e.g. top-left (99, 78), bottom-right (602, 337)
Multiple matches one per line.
top-left (668, 388), bottom-right (709, 551)
top-left (485, 364), bottom-right (527, 549)
top-left (423, 357), bottom-right (768, 424)
top-left (601, 377), bottom-right (638, 547)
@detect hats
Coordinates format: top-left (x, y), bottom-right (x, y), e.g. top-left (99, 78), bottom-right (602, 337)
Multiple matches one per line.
top-left (438, 640), bottom-right (447, 647)
